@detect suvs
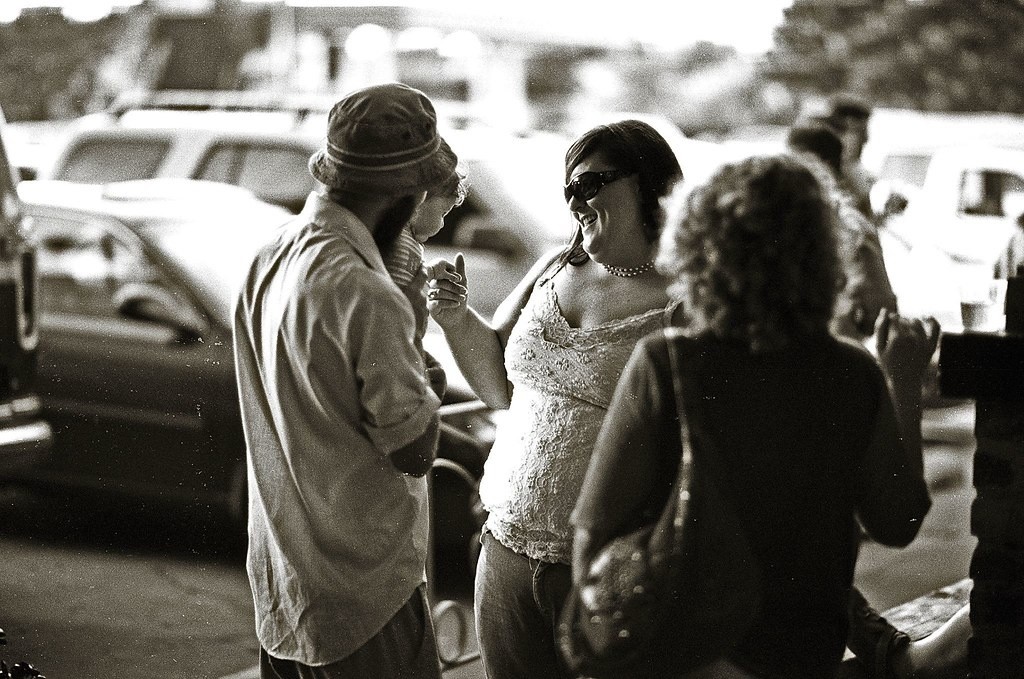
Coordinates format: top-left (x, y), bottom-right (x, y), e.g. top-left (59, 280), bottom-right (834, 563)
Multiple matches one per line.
top-left (39, 85), bottom-right (582, 322)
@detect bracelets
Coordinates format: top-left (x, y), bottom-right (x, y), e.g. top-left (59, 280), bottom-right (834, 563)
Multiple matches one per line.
top-left (431, 266), bottom-right (436, 279)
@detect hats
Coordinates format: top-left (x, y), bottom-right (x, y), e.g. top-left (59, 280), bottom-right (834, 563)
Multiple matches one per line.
top-left (308, 84), bottom-right (458, 197)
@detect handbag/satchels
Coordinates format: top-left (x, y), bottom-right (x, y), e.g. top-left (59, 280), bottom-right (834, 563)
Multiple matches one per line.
top-left (557, 327), bottom-right (768, 679)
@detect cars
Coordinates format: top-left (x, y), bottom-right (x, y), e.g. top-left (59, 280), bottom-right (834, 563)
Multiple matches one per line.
top-left (1, 181), bottom-right (505, 604)
top-left (863, 108), bottom-right (1023, 407)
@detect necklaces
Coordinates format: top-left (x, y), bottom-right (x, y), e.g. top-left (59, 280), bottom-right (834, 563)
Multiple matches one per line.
top-left (601, 258), bottom-right (655, 277)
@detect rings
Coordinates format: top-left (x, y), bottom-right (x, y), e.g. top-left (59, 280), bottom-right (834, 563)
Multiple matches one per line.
top-left (428, 289), bottom-right (440, 300)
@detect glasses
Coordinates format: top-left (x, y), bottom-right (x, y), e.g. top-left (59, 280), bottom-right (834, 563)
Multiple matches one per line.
top-left (563, 162), bottom-right (638, 204)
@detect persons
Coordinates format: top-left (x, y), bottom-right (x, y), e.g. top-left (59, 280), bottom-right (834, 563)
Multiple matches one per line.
top-left (426, 120), bottom-right (683, 679)
top-left (785, 92), bottom-right (908, 341)
top-left (567, 155), bottom-right (941, 679)
top-left (231, 83), bottom-right (466, 679)
